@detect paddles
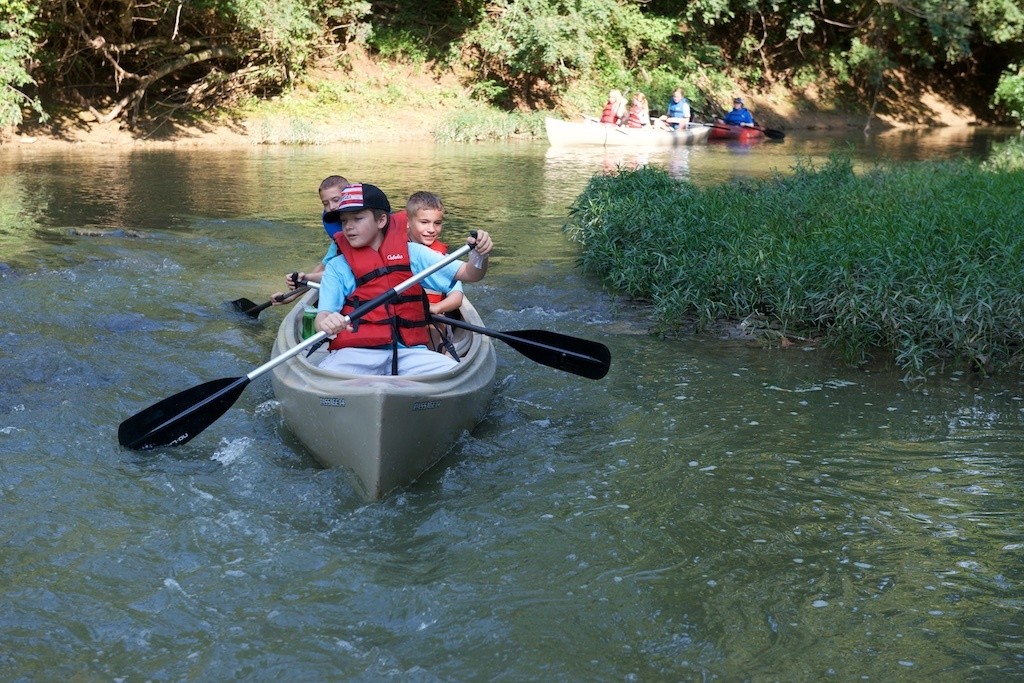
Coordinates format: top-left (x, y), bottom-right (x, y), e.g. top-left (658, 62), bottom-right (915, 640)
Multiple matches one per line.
top-left (578, 112), bottom-right (628, 136)
top-left (650, 116), bottom-right (731, 131)
top-left (690, 107), bottom-right (786, 139)
top-left (291, 272), bottom-right (611, 381)
top-left (118, 230), bottom-right (477, 451)
top-left (232, 286), bottom-right (309, 317)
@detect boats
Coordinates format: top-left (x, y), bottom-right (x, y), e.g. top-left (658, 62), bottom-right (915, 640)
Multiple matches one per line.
top-left (545, 116), bottom-right (714, 146)
top-left (271, 281), bottom-right (499, 513)
top-left (688, 123), bottom-right (765, 139)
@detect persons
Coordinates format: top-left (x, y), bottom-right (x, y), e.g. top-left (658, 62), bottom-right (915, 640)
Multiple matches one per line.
top-left (270, 176), bottom-right (352, 307)
top-left (661, 88), bottom-right (691, 129)
top-left (600, 89), bottom-right (629, 127)
top-left (324, 183), bottom-right (494, 377)
top-left (711, 97), bottom-right (754, 128)
top-left (617, 92), bottom-right (649, 128)
top-left (401, 191), bottom-right (464, 357)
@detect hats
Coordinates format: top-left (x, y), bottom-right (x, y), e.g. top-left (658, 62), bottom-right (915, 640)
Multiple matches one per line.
top-left (323, 182), bottom-right (391, 224)
top-left (733, 97), bottom-right (744, 105)
